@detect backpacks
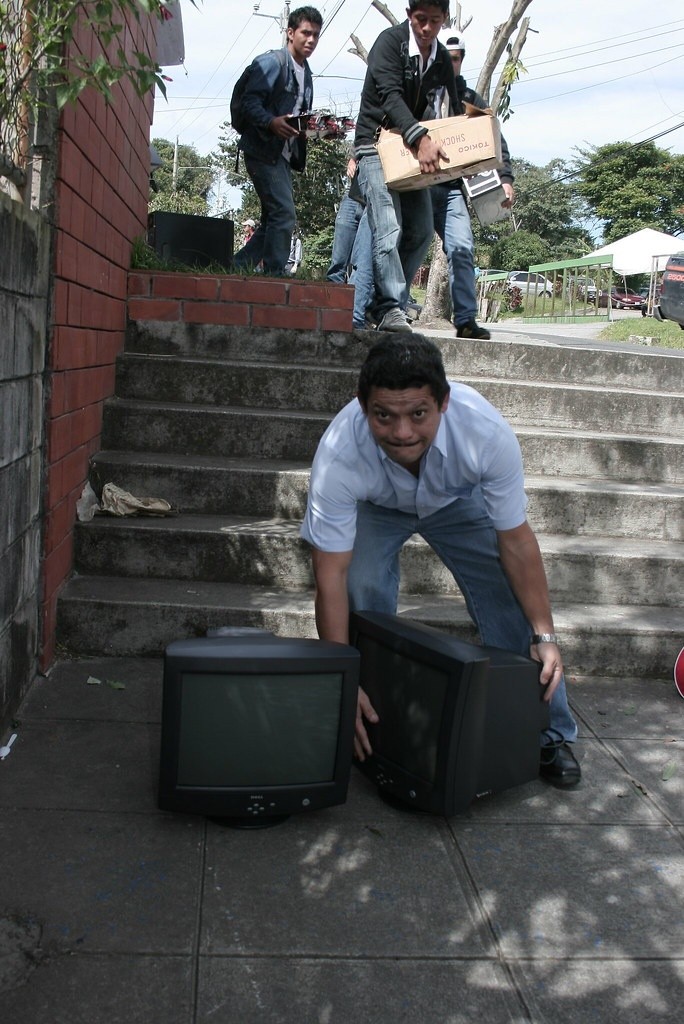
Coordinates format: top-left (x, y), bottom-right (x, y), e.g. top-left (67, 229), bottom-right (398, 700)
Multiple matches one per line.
top-left (230, 49), bottom-right (286, 135)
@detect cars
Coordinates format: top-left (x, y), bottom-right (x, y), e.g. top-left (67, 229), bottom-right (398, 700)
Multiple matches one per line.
top-left (568, 277), bottom-right (602, 302)
top-left (598, 285), bottom-right (648, 309)
top-left (502, 270), bottom-right (553, 298)
top-left (478, 269), bottom-right (508, 281)
top-left (642, 284), bottom-right (661, 317)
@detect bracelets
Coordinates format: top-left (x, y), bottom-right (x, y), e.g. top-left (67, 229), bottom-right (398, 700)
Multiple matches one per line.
top-left (530, 633), bottom-right (558, 644)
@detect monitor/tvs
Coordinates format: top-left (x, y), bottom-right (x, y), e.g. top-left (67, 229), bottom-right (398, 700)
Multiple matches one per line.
top-left (155, 624), bottom-right (360, 831)
top-left (345, 611), bottom-right (544, 819)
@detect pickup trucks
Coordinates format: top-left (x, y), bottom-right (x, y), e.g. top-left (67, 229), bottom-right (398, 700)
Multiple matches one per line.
top-left (653, 251), bottom-right (684, 329)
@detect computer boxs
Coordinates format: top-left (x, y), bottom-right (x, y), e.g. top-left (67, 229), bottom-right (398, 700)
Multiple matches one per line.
top-left (460, 168), bottom-right (512, 228)
top-left (149, 211), bottom-right (234, 273)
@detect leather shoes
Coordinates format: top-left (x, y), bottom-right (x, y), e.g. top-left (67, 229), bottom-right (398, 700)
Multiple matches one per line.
top-left (540, 741), bottom-right (581, 785)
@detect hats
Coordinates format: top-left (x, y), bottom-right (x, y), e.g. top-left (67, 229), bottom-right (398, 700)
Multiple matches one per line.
top-left (437, 29), bottom-right (465, 50)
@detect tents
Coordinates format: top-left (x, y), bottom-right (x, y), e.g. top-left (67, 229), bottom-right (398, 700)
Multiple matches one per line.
top-left (568, 228), bottom-right (684, 315)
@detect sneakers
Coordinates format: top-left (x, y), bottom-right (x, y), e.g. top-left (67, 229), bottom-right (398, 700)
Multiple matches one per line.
top-left (456, 317), bottom-right (490, 340)
top-left (378, 307), bottom-right (413, 332)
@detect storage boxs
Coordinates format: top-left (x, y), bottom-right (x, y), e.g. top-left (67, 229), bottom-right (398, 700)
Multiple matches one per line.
top-left (372, 100), bottom-right (502, 192)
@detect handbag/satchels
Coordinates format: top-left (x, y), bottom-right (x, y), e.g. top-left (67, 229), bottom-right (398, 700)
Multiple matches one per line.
top-left (348, 168), bottom-right (366, 206)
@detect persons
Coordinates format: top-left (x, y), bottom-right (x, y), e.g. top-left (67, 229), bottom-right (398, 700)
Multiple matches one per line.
top-left (230, 0), bottom-right (515, 340)
top-left (299, 333), bottom-right (582, 790)
